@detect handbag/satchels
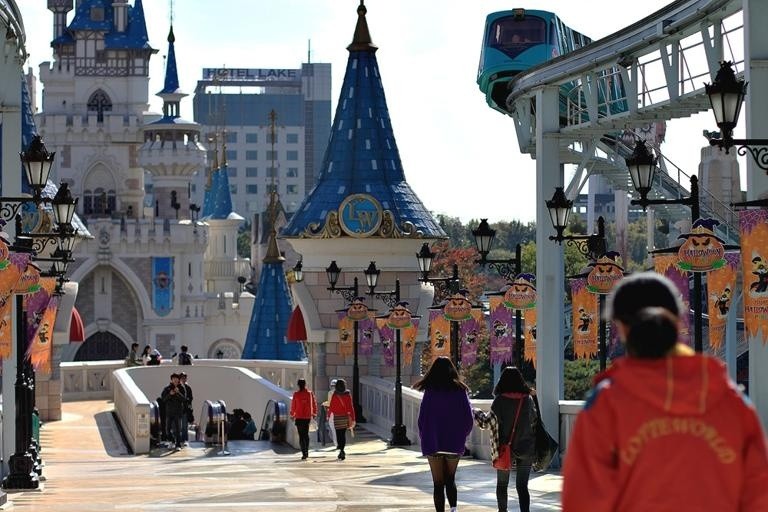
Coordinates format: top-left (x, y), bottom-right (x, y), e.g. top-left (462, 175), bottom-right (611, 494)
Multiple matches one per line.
top-left (531, 420), bottom-right (559, 473)
top-left (490, 439), bottom-right (511, 471)
top-left (347, 414), bottom-right (354, 429)
top-left (309, 416), bottom-right (319, 433)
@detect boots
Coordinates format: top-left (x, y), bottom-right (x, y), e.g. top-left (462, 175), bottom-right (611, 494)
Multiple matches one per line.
top-left (299, 437), bottom-right (310, 460)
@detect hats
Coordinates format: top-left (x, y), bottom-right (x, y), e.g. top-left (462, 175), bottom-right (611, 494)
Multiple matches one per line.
top-left (329, 379), bottom-right (339, 386)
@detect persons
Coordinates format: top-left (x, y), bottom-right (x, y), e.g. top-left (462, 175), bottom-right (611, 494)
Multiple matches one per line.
top-left (327, 379), bottom-right (356, 460)
top-left (141, 344), bottom-right (162, 365)
top-left (146, 349), bottom-right (160, 365)
top-left (226, 407), bottom-right (257, 440)
top-left (410, 355), bottom-right (475, 511)
top-left (470, 365), bottom-right (538, 511)
top-left (160, 372), bottom-right (187, 450)
top-left (128, 342), bottom-right (144, 367)
top-left (320, 378), bottom-right (339, 448)
top-left (171, 352), bottom-right (177, 364)
top-left (178, 371), bottom-right (193, 448)
top-left (177, 344), bottom-right (193, 365)
top-left (559, 272), bottom-right (768, 512)
top-left (289, 378), bottom-right (317, 461)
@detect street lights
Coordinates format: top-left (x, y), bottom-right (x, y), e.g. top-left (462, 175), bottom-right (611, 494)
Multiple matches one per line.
top-left (280, 247), bottom-right (307, 291)
top-left (0, 134), bottom-right (77, 490)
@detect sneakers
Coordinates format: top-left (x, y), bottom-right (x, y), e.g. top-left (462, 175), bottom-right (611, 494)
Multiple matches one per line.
top-left (159, 439), bottom-right (188, 451)
top-left (338, 451), bottom-right (347, 460)
